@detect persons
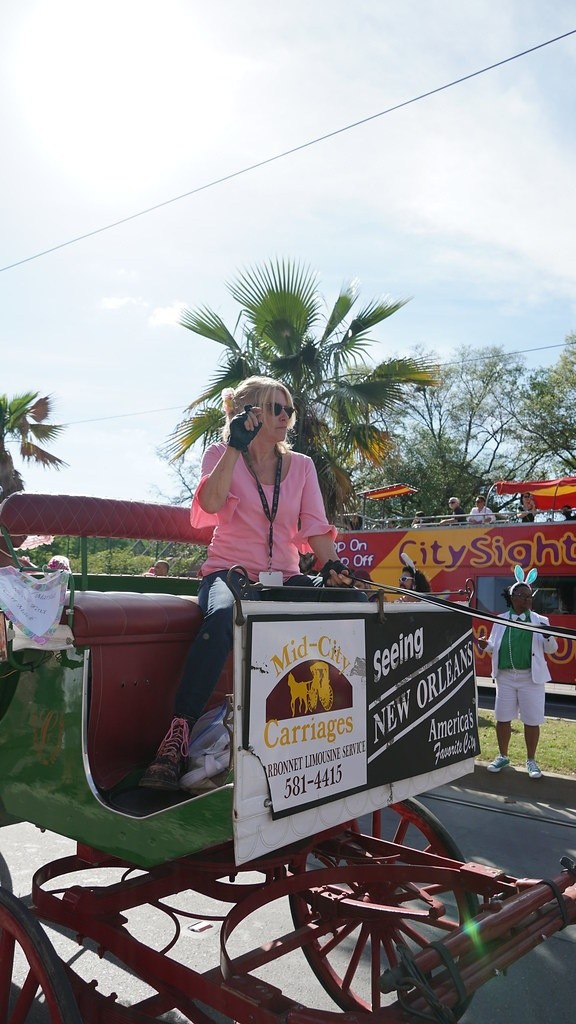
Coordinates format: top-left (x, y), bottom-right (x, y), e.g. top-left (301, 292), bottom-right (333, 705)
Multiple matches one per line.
top-left (354, 570), bottom-right (380, 602)
top-left (439, 497), bottom-right (467, 526)
top-left (477, 580), bottom-right (558, 779)
top-left (47, 555), bottom-right (73, 573)
top-left (397, 565), bottom-right (430, 602)
top-left (135, 374), bottom-right (368, 793)
top-left (517, 492), bottom-right (537, 522)
top-left (467, 497), bottom-right (496, 525)
top-left (154, 559), bottom-right (169, 575)
top-left (346, 515), bottom-right (362, 530)
top-left (385, 518), bottom-right (401, 528)
top-left (410, 510), bottom-right (428, 527)
top-left (561, 505), bottom-right (576, 520)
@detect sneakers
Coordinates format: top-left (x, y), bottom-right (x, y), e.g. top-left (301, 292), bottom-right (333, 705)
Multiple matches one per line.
top-left (526, 760), bottom-right (541, 778)
top-left (487, 754), bottom-right (510, 772)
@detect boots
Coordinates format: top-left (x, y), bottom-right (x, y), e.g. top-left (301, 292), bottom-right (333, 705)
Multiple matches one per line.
top-left (138, 713), bottom-right (196, 791)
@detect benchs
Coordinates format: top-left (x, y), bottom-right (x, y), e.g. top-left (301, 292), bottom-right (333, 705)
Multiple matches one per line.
top-left (0, 494), bottom-right (233, 788)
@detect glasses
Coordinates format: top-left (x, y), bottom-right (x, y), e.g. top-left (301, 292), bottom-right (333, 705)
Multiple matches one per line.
top-left (512, 593), bottom-right (534, 600)
top-left (448, 502), bottom-right (456, 504)
top-left (524, 495), bottom-right (530, 498)
top-left (399, 576), bottom-right (413, 582)
top-left (260, 402), bottom-right (293, 419)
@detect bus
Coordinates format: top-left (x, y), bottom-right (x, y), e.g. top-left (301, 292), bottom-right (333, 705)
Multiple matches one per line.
top-left (298, 477), bottom-right (576, 699)
